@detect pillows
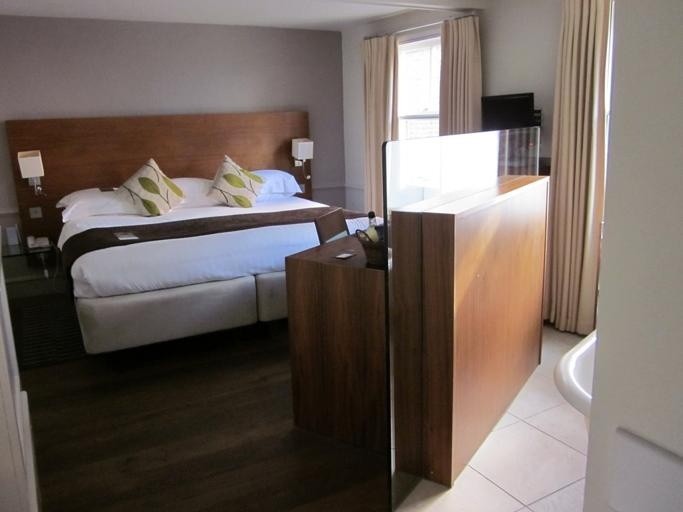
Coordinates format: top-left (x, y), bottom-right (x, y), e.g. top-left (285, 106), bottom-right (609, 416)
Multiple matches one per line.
top-left (55, 154), bottom-right (300, 224)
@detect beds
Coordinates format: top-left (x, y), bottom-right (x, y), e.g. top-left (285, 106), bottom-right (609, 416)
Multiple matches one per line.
top-left (53, 196), bottom-right (385, 360)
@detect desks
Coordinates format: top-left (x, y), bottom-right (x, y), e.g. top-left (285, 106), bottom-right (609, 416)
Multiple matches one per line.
top-left (1, 242), bottom-right (54, 281)
top-left (283, 227), bottom-right (386, 454)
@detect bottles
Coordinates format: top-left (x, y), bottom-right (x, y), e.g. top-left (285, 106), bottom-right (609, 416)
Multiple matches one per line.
top-left (365, 211), bottom-right (379, 242)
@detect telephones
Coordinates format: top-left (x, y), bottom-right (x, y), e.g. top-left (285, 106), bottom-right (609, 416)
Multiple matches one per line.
top-left (27, 236), bottom-right (51, 249)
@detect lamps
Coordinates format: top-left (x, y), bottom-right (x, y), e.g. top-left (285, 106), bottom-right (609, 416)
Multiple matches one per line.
top-left (17, 150), bottom-right (45, 186)
top-left (290, 136), bottom-right (313, 166)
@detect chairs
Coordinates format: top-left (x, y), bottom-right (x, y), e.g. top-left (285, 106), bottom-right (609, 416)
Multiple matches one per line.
top-left (314, 207), bottom-right (350, 248)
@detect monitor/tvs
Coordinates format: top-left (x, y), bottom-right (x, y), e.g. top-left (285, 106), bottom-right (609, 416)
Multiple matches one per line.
top-left (481, 92), bottom-right (534, 131)
